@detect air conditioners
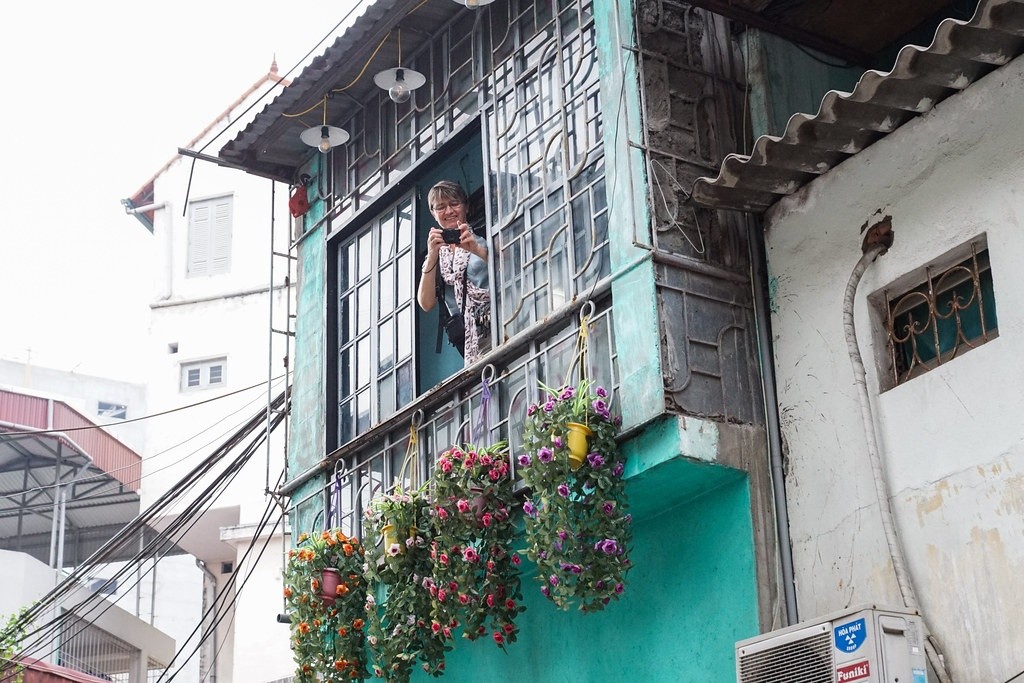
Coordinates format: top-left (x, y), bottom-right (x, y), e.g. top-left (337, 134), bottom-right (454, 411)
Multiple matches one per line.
top-left (733, 599), bottom-right (931, 682)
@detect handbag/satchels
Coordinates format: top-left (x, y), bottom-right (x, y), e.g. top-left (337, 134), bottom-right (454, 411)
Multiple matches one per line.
top-left (444, 314), bottom-right (467, 358)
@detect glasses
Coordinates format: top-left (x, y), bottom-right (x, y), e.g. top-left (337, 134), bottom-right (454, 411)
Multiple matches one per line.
top-left (432, 200), bottom-right (465, 213)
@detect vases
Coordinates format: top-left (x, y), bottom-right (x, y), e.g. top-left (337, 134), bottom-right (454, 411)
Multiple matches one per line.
top-left (468, 487), bottom-right (492, 528)
top-left (316, 566), bottom-right (342, 604)
top-left (381, 522), bottom-right (419, 565)
top-left (565, 421), bottom-right (595, 473)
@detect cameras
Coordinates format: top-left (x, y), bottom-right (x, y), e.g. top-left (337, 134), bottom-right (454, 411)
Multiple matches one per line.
top-left (441, 228), bottom-right (461, 244)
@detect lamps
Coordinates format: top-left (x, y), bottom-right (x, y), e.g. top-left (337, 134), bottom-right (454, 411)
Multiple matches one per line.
top-left (300, 93), bottom-right (350, 155)
top-left (454, 0), bottom-right (498, 10)
top-left (373, 27), bottom-right (428, 105)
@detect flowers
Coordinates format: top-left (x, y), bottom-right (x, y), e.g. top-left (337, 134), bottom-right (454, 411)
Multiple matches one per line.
top-left (284, 528), bottom-right (367, 683)
top-left (516, 376), bottom-right (638, 616)
top-left (360, 485), bottom-right (455, 683)
top-left (425, 439), bottom-right (526, 655)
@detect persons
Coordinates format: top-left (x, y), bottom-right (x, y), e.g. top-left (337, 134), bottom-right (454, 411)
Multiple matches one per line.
top-left (417, 179), bottom-right (489, 361)
